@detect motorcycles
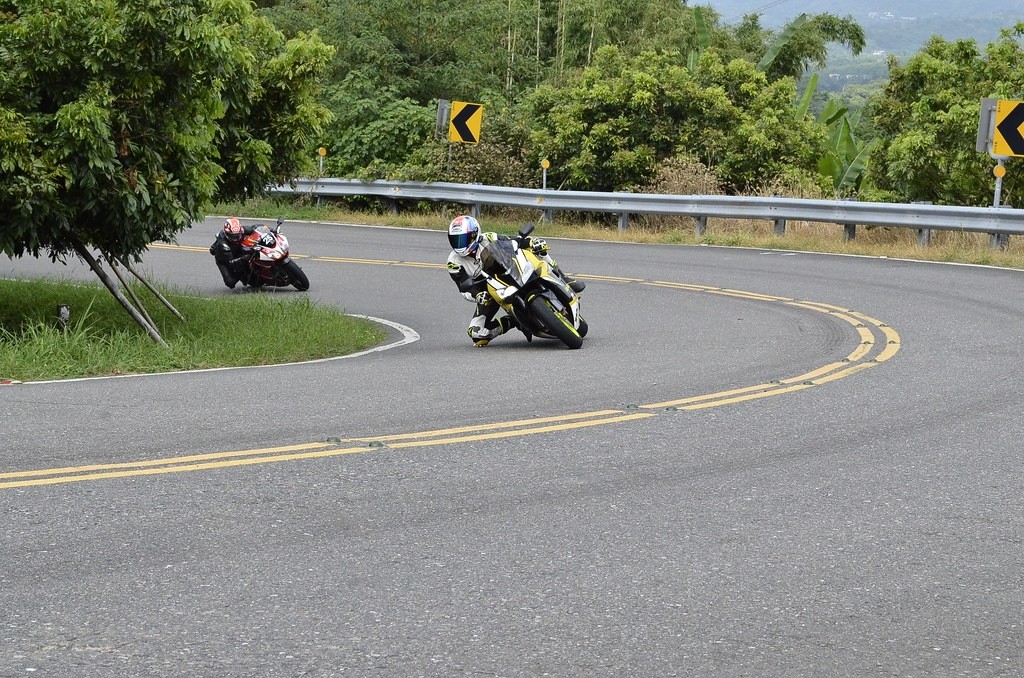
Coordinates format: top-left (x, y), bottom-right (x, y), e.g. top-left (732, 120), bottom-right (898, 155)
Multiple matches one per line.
top-left (452, 223), bottom-right (585, 349)
top-left (215, 216), bottom-right (310, 291)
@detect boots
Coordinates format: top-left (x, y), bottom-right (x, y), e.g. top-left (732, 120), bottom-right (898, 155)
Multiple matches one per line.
top-left (551, 260), bottom-right (586, 292)
top-left (494, 314), bottom-right (532, 342)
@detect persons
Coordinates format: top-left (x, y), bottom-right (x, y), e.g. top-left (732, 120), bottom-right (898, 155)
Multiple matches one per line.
top-left (448, 215), bottom-right (587, 344)
top-left (214, 217), bottom-right (268, 288)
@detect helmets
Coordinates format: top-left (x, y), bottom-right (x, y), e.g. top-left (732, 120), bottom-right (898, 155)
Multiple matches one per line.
top-left (448, 215), bottom-right (482, 258)
top-left (224, 218), bottom-right (241, 242)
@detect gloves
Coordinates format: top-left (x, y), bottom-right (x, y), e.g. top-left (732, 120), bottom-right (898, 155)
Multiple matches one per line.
top-left (475, 291), bottom-right (492, 304)
top-left (270, 227), bottom-right (278, 234)
top-left (241, 254), bottom-right (252, 261)
top-left (529, 238), bottom-right (547, 250)
